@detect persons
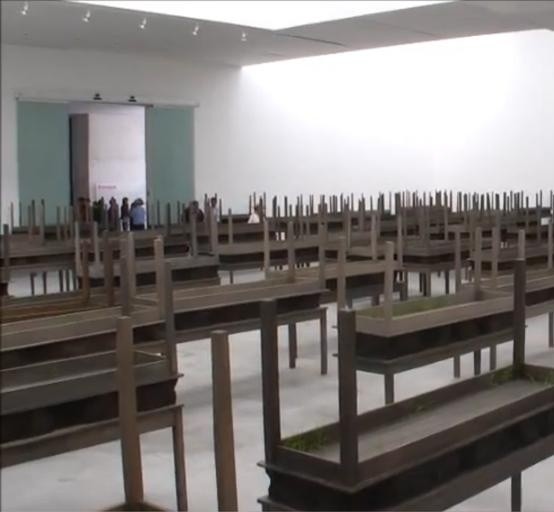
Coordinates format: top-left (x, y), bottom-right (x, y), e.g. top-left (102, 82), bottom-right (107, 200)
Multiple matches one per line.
top-left (182, 201), bottom-right (204, 222)
top-left (70, 195), bottom-right (147, 234)
top-left (205, 196), bottom-right (219, 222)
top-left (246, 205), bottom-right (266, 224)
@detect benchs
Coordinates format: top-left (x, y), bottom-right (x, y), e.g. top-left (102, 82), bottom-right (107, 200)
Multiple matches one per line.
top-left (0, 185), bottom-right (553, 512)
